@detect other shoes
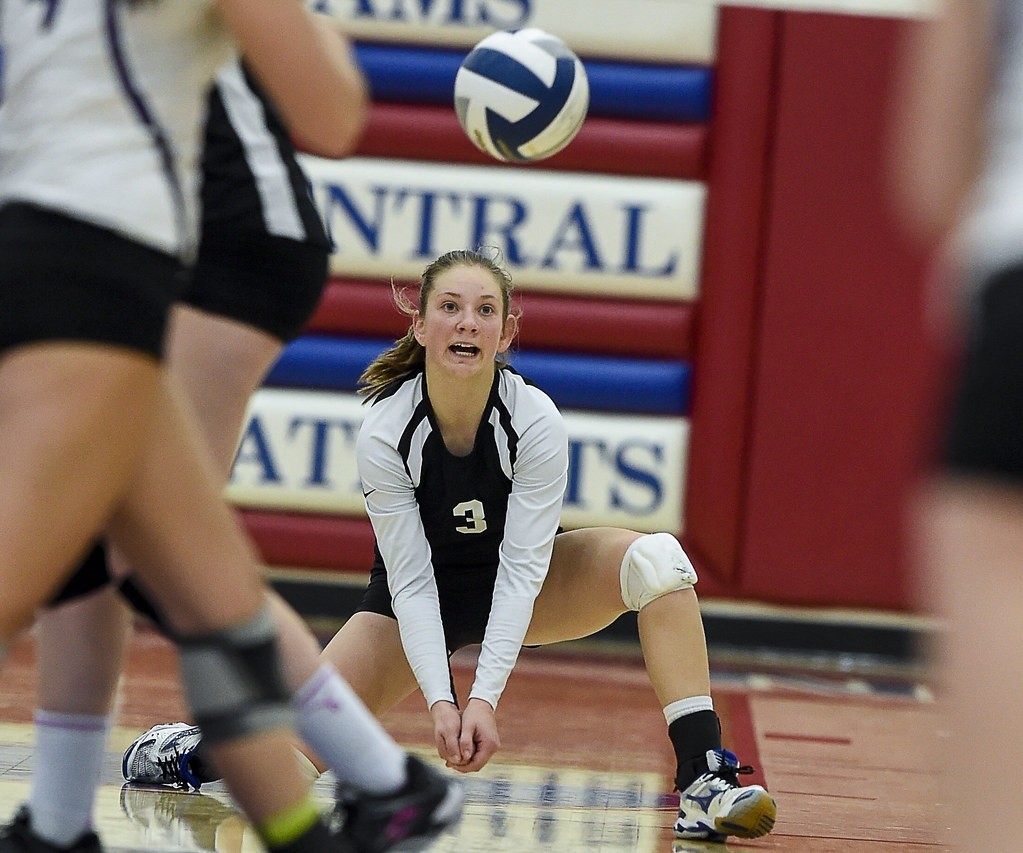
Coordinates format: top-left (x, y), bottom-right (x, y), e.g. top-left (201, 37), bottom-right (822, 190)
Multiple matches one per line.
top-left (0, 806), bottom-right (102, 853)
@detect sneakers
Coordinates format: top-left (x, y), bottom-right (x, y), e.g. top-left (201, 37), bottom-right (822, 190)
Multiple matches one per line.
top-left (329, 751), bottom-right (466, 853)
top-left (121, 721), bottom-right (225, 793)
top-left (673, 748), bottom-right (777, 844)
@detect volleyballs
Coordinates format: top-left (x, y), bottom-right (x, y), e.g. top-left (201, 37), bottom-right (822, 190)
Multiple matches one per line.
top-left (454, 28), bottom-right (590, 164)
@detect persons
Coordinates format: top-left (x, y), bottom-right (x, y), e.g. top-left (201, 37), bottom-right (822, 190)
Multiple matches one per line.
top-left (0, 0), bottom-right (470, 853)
top-left (120, 252), bottom-right (775, 840)
top-left (891, 0), bottom-right (1023, 853)
